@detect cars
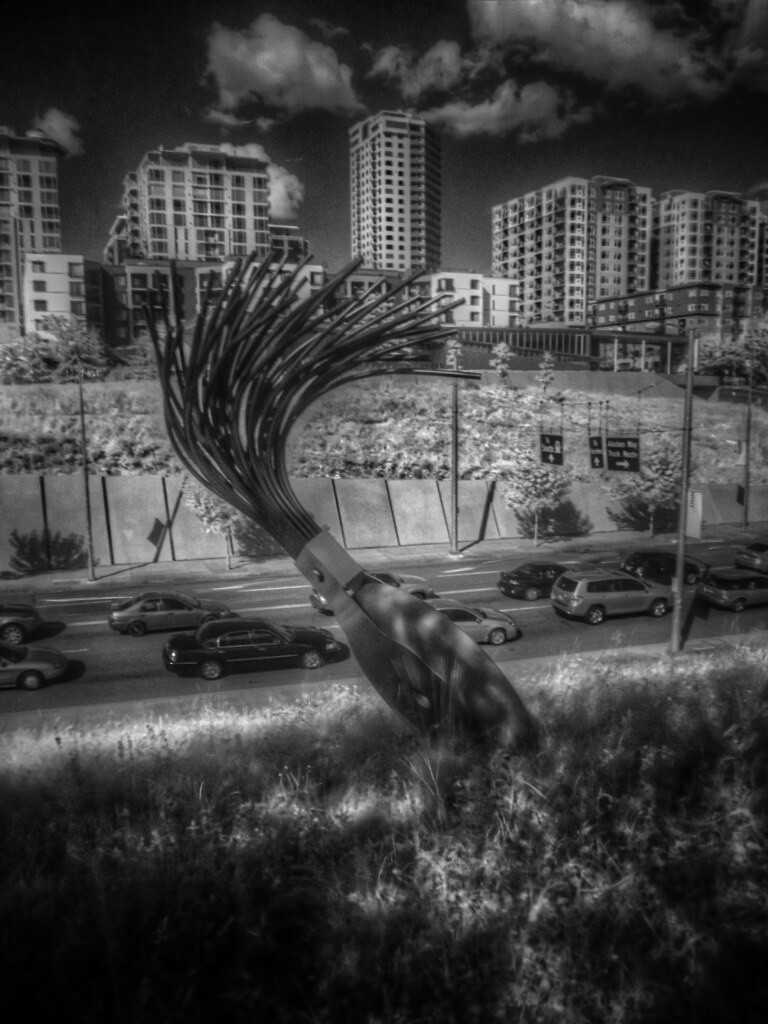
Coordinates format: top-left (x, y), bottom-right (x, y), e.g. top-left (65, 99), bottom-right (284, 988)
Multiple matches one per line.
top-left (550, 565), bottom-right (674, 625)
top-left (735, 541), bottom-right (768, 575)
top-left (0, 641), bottom-right (70, 692)
top-left (309, 563), bottom-right (434, 613)
top-left (618, 547), bottom-right (711, 606)
top-left (424, 597), bottom-right (520, 647)
top-left (109, 589), bottom-right (231, 638)
top-left (497, 560), bottom-right (575, 602)
top-left (162, 616), bottom-right (341, 681)
top-left (0, 602), bottom-right (45, 646)
top-left (698, 567), bottom-right (768, 613)
